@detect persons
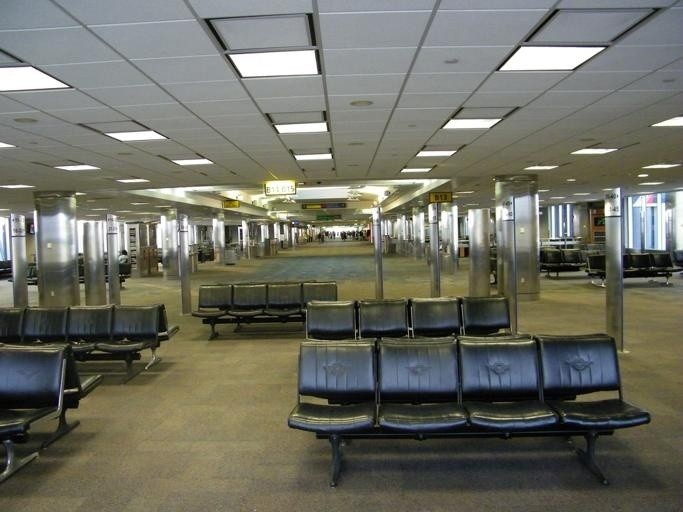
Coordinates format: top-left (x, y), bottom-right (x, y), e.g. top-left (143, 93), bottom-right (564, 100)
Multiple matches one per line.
top-left (304, 229), bottom-right (366, 243)
top-left (119, 250), bottom-right (128, 263)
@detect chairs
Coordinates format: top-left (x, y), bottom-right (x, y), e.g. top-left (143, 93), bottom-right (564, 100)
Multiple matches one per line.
top-left (0, 306), bottom-right (180, 386)
top-left (25, 264), bottom-right (132, 288)
top-left (0, 343), bottom-right (103, 483)
top-left (306, 295), bottom-right (511, 338)
top-left (192, 281), bottom-right (339, 342)
top-left (489, 248), bottom-right (683, 287)
